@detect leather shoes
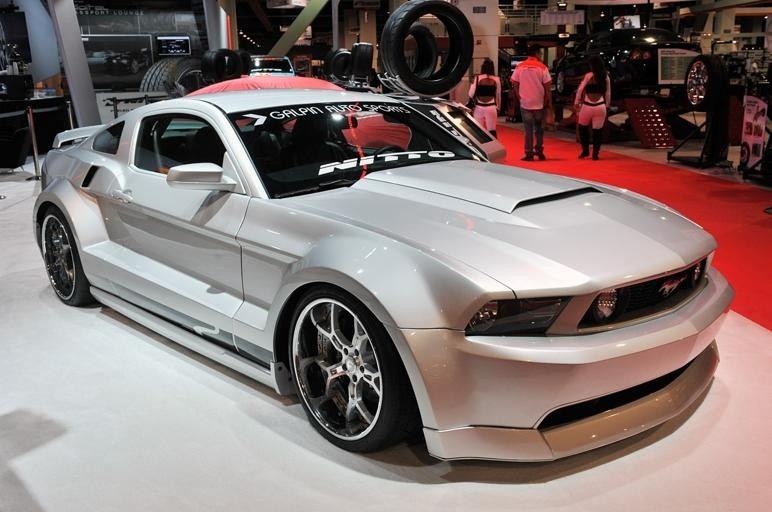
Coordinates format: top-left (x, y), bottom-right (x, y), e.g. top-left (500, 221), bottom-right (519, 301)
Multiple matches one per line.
top-left (520, 152), bottom-right (545, 161)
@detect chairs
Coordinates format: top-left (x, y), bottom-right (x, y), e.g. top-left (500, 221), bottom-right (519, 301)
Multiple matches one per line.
top-left (184, 126), bottom-right (225, 166)
top-left (274, 113), bottom-right (347, 170)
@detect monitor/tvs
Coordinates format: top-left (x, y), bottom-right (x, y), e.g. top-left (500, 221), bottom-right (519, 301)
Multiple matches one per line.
top-left (81, 34), bottom-right (154, 92)
top-left (613, 14), bottom-right (641, 29)
top-left (510, 55), bottom-right (528, 72)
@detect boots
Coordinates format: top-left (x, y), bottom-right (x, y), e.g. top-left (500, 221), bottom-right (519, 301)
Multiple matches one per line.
top-left (489, 130), bottom-right (496, 137)
top-left (578, 123), bottom-right (602, 160)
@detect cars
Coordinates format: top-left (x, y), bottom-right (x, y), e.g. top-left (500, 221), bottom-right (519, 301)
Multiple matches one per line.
top-left (553, 26), bottom-right (703, 100)
top-left (251, 54), bottom-right (296, 77)
top-left (28, 87), bottom-right (735, 464)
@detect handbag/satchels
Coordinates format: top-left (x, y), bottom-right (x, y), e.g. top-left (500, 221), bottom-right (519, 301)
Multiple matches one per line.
top-left (466, 98), bottom-right (475, 109)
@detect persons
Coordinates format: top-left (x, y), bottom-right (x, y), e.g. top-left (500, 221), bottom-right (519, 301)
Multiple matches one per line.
top-left (469, 57), bottom-right (503, 140)
top-left (573, 57), bottom-right (611, 160)
top-left (510, 44), bottom-right (552, 160)
